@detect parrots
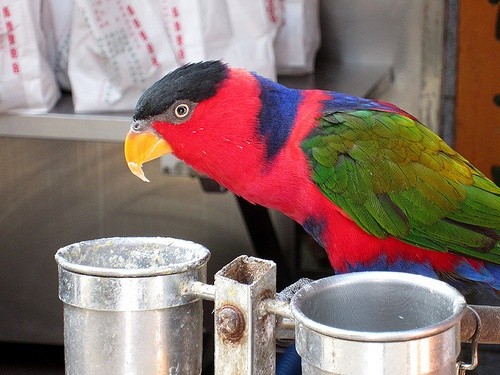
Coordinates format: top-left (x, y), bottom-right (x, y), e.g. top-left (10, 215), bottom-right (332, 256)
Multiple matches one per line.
top-left (122, 59), bottom-right (500, 301)
